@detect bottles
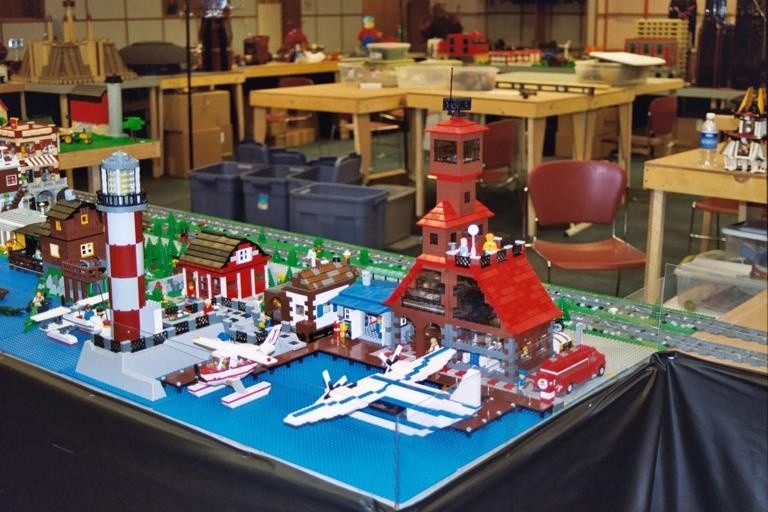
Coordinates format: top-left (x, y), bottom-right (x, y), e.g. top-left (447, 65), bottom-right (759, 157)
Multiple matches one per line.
top-left (697, 112), bottom-right (718, 168)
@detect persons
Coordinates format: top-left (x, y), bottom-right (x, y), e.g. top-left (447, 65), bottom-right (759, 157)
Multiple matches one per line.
top-left (1, 114), bottom-right (348, 345)
top-left (376, 147), bottom-right (532, 361)
top-left (415, 2), bottom-right (463, 44)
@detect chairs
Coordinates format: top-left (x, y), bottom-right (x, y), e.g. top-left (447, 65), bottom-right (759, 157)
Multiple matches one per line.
top-left (526, 160), bottom-right (646, 296)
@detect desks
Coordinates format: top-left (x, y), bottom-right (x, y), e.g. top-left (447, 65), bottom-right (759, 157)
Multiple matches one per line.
top-left (643, 141), bottom-right (768, 375)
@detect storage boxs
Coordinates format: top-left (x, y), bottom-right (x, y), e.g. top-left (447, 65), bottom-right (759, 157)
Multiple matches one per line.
top-left (674, 220), bottom-right (768, 318)
top-left (286, 183), bottom-right (417, 251)
